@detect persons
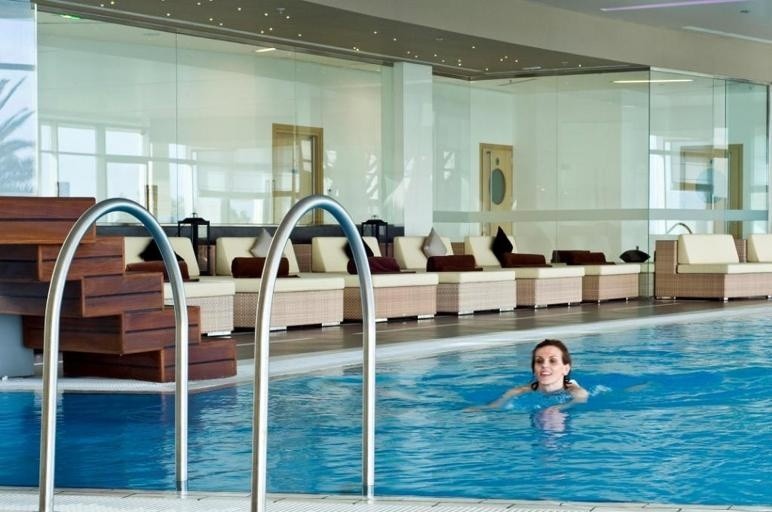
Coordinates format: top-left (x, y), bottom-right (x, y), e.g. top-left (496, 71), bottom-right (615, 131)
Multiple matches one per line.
top-left (530, 406), bottom-right (569, 449)
top-left (464, 339), bottom-right (589, 413)
